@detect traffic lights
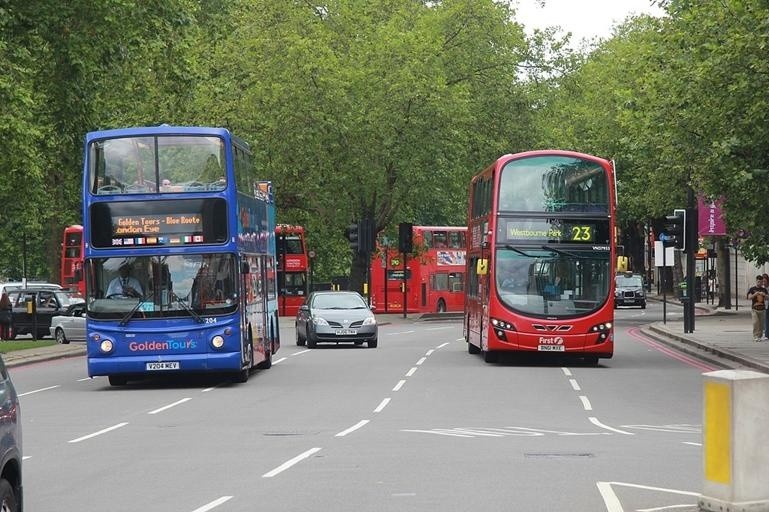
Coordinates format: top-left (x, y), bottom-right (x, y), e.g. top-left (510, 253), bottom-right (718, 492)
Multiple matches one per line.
top-left (348, 218), bottom-right (372, 256)
top-left (663, 208), bottom-right (699, 252)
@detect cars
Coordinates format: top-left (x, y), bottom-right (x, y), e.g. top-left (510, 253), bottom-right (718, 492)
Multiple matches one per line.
top-left (0, 282), bottom-right (88, 344)
top-left (294, 289), bottom-right (378, 347)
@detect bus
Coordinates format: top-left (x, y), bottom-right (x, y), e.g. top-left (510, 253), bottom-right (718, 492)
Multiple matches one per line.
top-left (462, 149), bottom-right (629, 368)
top-left (368, 222), bottom-right (468, 314)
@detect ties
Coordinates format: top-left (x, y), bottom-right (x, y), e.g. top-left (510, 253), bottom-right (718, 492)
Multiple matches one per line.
top-left (123, 279), bottom-right (126, 296)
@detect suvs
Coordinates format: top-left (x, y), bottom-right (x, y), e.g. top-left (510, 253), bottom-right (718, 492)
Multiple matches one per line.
top-left (614, 274), bottom-right (647, 309)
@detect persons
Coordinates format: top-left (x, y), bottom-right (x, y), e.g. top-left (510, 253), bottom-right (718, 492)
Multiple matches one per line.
top-left (0, 293), bottom-right (13, 342)
top-left (104, 263), bottom-right (144, 300)
top-left (500, 268), bottom-right (529, 287)
top-left (747, 273), bottom-right (769, 342)
top-left (161, 179), bottom-right (171, 187)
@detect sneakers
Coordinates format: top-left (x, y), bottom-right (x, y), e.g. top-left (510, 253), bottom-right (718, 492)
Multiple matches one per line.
top-left (765, 337), bottom-right (768, 339)
